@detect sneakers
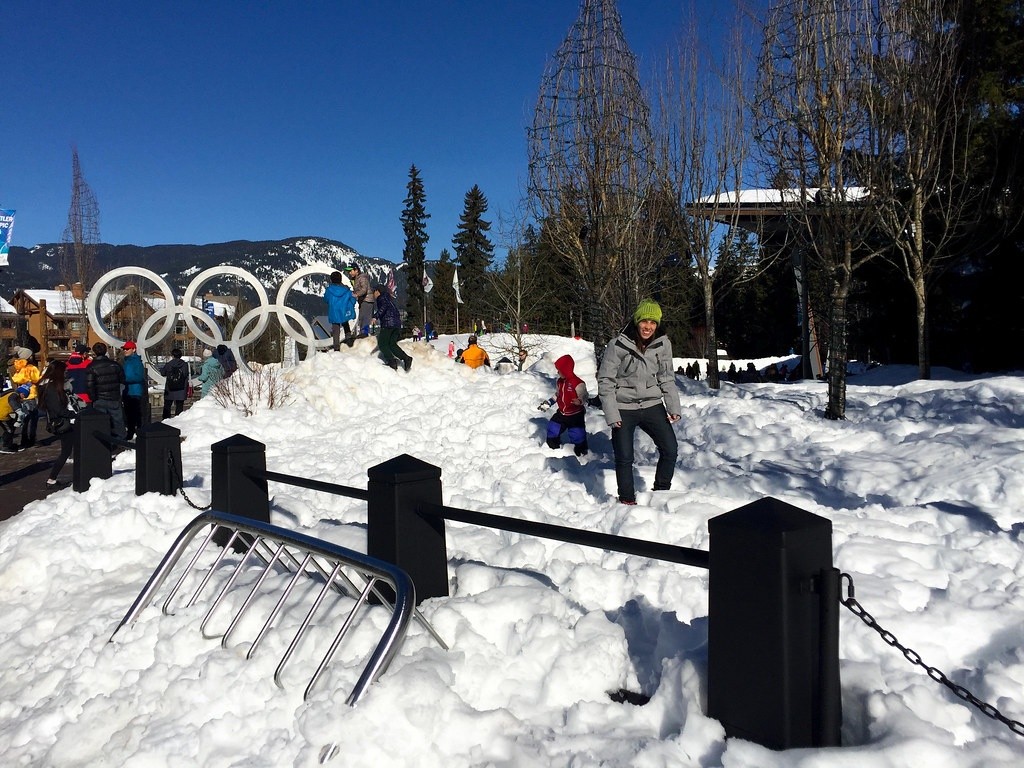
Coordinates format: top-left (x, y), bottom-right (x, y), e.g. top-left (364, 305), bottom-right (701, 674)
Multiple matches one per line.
top-left (383, 362), bottom-right (398, 369)
top-left (404, 356), bottom-right (412, 371)
top-left (46, 481), bottom-right (65, 489)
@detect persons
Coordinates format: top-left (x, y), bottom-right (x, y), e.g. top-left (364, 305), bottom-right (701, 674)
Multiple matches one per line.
top-left (412, 317), bottom-right (528, 371)
top-left (674, 360), bottom-right (792, 384)
top-left (537, 354), bottom-right (589, 457)
top-left (597, 297), bottom-right (682, 507)
top-left (345, 263), bottom-right (374, 339)
top-left (324, 271), bottom-right (356, 352)
top-left (369, 284), bottom-right (413, 373)
top-left (0, 330), bottom-right (237, 488)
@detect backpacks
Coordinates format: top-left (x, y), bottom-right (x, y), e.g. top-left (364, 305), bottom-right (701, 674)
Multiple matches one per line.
top-left (167, 367), bottom-right (185, 391)
top-left (63, 369), bottom-right (88, 393)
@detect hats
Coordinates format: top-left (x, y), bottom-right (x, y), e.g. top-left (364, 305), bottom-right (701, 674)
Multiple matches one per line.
top-left (75, 344), bottom-right (91, 352)
top-left (17, 383), bottom-right (32, 398)
top-left (14, 346), bottom-right (32, 359)
top-left (634, 297), bottom-right (662, 328)
top-left (217, 345), bottom-right (227, 355)
top-left (344, 263), bottom-right (359, 270)
top-left (203, 349), bottom-right (212, 357)
top-left (121, 341), bottom-right (136, 348)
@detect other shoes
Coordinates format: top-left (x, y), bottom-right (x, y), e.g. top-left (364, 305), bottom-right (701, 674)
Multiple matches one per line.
top-left (358, 333), bottom-right (368, 337)
top-left (622, 500), bottom-right (637, 505)
top-left (0, 445), bottom-right (25, 454)
top-left (20, 443), bottom-right (41, 449)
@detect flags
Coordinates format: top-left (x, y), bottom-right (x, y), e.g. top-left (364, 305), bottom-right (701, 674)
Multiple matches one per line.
top-left (387, 271), bottom-right (397, 299)
top-left (452, 270), bottom-right (464, 303)
top-left (422, 270), bottom-right (433, 293)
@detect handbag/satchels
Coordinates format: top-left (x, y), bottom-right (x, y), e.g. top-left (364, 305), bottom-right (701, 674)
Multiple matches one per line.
top-left (45, 390), bottom-right (71, 435)
top-left (187, 387), bottom-right (194, 398)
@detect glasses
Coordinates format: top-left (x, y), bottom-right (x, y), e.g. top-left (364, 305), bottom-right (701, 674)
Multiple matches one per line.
top-left (123, 348), bottom-right (129, 351)
top-left (519, 353), bottom-right (525, 356)
